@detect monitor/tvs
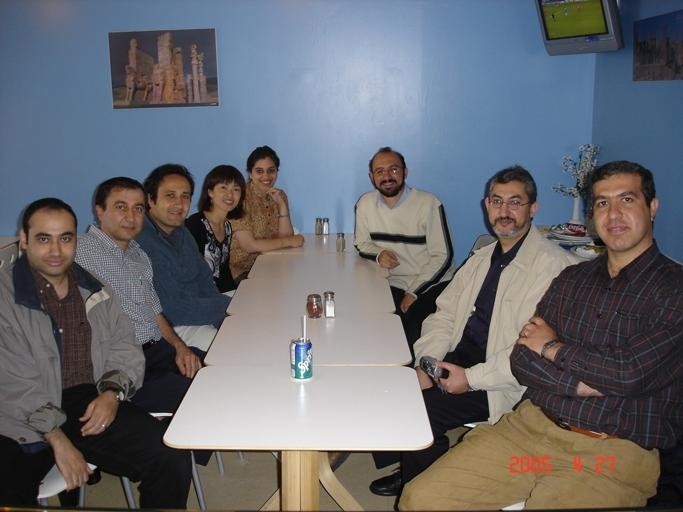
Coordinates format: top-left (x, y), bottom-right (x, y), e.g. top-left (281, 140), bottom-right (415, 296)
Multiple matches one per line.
top-left (534, 0), bottom-right (625, 57)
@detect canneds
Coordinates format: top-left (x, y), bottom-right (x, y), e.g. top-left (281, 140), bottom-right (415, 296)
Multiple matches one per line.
top-left (288, 338), bottom-right (314, 381)
top-left (565, 223), bottom-right (587, 237)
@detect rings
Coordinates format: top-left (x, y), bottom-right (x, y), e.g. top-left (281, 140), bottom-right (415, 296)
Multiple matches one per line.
top-left (524, 330), bottom-right (528, 339)
top-left (73, 479), bottom-right (79, 483)
top-left (101, 425), bottom-right (107, 428)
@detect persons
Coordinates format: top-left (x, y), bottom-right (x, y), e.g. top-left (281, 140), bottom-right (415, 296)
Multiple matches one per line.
top-left (186, 165), bottom-right (247, 298)
top-left (396, 159), bottom-right (682, 511)
top-left (353, 147), bottom-right (457, 357)
top-left (133, 164), bottom-right (233, 352)
top-left (0, 198), bottom-right (192, 512)
top-left (229, 145), bottom-right (304, 288)
top-left (72, 176), bottom-right (213, 467)
top-left (370, 165), bottom-right (583, 496)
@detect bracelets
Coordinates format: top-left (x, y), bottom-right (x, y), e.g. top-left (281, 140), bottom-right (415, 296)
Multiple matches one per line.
top-left (280, 239), bottom-right (284, 249)
top-left (540, 338), bottom-right (558, 359)
top-left (279, 215), bottom-right (289, 217)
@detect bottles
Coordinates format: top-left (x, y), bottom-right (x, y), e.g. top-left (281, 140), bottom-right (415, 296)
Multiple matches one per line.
top-left (335, 232), bottom-right (346, 254)
top-left (314, 218), bottom-right (322, 235)
top-left (322, 291), bottom-right (334, 318)
top-left (306, 294), bottom-right (323, 319)
top-left (322, 218), bottom-right (328, 234)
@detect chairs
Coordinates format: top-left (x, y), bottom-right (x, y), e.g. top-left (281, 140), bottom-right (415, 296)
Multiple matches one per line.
top-left (150, 413), bottom-right (224, 510)
top-left (37, 459), bottom-right (137, 509)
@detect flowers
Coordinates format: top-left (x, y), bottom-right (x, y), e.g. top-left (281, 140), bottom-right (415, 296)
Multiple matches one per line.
top-left (552, 143), bottom-right (599, 198)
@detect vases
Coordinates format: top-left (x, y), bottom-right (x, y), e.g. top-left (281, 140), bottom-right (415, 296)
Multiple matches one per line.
top-left (564, 196), bottom-right (587, 237)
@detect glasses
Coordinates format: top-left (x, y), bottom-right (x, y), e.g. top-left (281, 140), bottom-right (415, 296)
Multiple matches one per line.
top-left (489, 200), bottom-right (529, 210)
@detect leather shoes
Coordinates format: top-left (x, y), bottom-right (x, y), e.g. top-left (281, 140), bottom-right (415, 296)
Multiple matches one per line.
top-left (370, 472), bottom-right (404, 495)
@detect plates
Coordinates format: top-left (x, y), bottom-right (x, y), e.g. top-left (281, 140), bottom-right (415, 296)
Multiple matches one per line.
top-left (570, 246), bottom-right (598, 259)
top-left (550, 231), bottom-right (592, 241)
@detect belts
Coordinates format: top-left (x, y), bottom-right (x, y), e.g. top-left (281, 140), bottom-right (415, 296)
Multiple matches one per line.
top-left (538, 405), bottom-right (621, 438)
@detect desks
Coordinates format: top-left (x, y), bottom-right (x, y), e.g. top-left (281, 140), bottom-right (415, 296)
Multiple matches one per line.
top-left (203, 313), bottom-right (413, 365)
top-left (247, 255), bottom-right (391, 278)
top-left (225, 278), bottom-right (397, 313)
top-left (162, 365), bottom-right (434, 512)
top-left (260, 233), bottom-right (359, 255)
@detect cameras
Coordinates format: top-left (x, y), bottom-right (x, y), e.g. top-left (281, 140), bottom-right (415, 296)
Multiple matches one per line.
top-left (421, 355), bottom-right (444, 379)
